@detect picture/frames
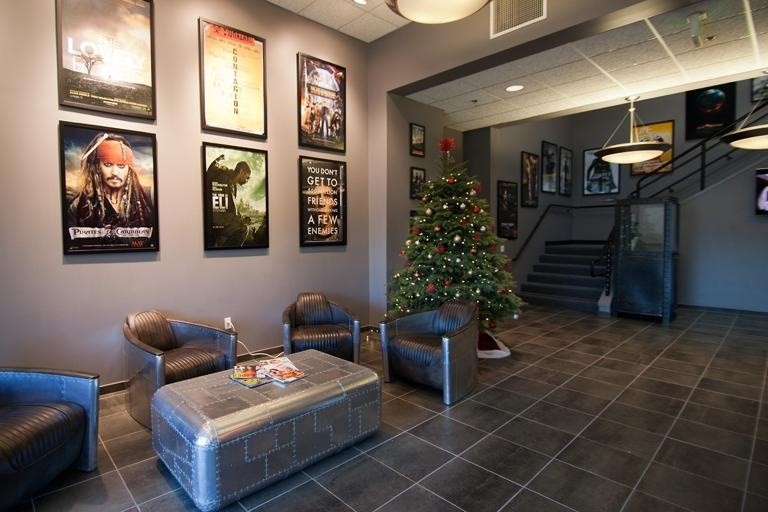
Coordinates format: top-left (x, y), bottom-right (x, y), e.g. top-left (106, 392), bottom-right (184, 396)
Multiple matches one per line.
top-left (298, 154), bottom-right (348, 248)
top-left (685, 81), bottom-right (737, 141)
top-left (58, 119), bottom-right (160, 257)
top-left (296, 50), bottom-right (347, 155)
top-left (201, 140), bottom-right (270, 252)
top-left (629, 119), bottom-right (675, 178)
top-left (408, 121), bottom-right (426, 158)
top-left (750, 76), bottom-right (768, 103)
top-left (409, 165), bottom-right (426, 200)
top-left (197, 15), bottom-right (269, 141)
top-left (496, 140), bottom-right (622, 242)
top-left (53, 1), bottom-right (157, 123)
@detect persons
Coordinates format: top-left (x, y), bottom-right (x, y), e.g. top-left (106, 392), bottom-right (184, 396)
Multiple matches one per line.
top-left (299, 71), bottom-right (345, 141)
top-left (544, 147), bottom-right (555, 191)
top-left (586, 152), bottom-right (617, 193)
top-left (303, 178), bottom-right (344, 241)
top-left (208, 154), bottom-right (252, 247)
top-left (67, 132), bottom-right (154, 247)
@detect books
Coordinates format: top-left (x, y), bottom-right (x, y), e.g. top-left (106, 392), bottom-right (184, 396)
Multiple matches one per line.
top-left (228, 355), bottom-right (307, 389)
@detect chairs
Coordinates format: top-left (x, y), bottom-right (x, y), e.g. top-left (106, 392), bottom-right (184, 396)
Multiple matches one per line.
top-left (378, 299), bottom-right (482, 407)
top-left (0, 362), bottom-right (100, 510)
top-left (281, 290), bottom-right (361, 362)
top-left (121, 306), bottom-right (237, 430)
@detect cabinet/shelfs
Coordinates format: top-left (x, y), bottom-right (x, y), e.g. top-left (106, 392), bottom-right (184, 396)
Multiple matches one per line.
top-left (612, 197), bottom-right (680, 325)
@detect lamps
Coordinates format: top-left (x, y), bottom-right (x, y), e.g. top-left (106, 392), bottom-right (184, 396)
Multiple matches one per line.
top-left (719, 75), bottom-right (767, 154)
top-left (382, 1), bottom-right (493, 28)
top-left (593, 93), bottom-right (672, 167)
